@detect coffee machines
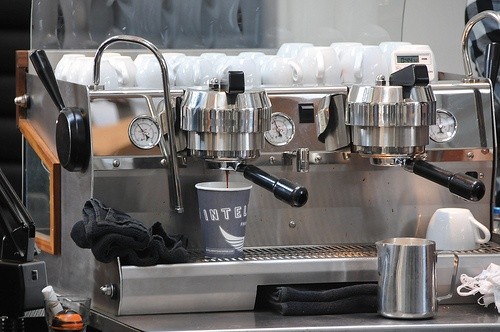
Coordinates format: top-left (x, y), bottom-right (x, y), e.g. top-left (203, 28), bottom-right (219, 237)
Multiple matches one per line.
top-left (0, 0), bottom-right (500, 315)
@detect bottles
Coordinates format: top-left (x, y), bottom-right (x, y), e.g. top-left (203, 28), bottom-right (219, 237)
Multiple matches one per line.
top-left (492, 207), bottom-right (500, 235)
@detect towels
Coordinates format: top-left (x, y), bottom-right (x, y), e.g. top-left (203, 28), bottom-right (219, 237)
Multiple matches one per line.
top-left (81, 196), bottom-right (189, 259)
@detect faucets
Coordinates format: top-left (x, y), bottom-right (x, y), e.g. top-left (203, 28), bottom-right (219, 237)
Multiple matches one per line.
top-left (401, 156), bottom-right (486, 203)
top-left (205, 158), bottom-right (309, 208)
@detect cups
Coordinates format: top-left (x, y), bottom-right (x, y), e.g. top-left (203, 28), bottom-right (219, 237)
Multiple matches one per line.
top-left (194, 181), bottom-right (252, 258)
top-left (44, 296), bottom-right (91, 332)
top-left (375, 237), bottom-right (459, 319)
top-left (425, 208), bottom-right (490, 251)
